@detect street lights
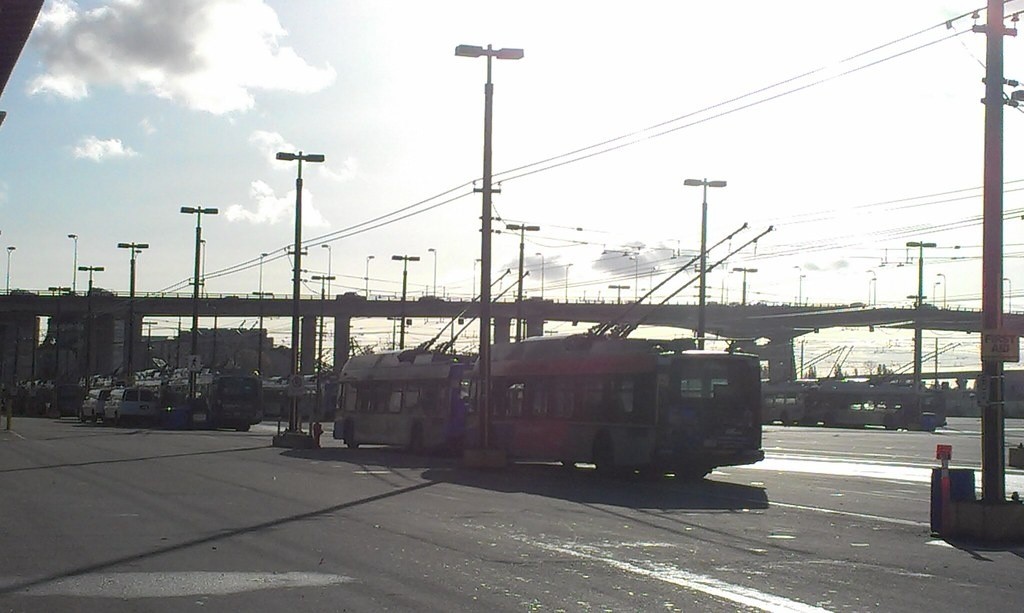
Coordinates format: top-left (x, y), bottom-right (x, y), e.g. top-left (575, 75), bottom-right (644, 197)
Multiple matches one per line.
top-left (366, 256), bottom-right (375, 299)
top-left (454, 44), bottom-right (525, 461)
top-left (311, 275), bottom-right (337, 447)
top-left (391, 255), bottom-right (420, 349)
top-left (506, 223), bottom-right (541, 342)
top-left (905, 241), bottom-right (937, 387)
top-left (607, 285), bottom-right (630, 305)
top-left (565, 263), bottom-right (574, 304)
top-left (117, 242), bottom-right (149, 388)
top-left (180, 206), bottom-right (218, 430)
top-left (428, 248), bottom-right (437, 297)
top-left (684, 178), bottom-right (728, 350)
top-left (6, 246), bottom-right (16, 295)
top-left (78, 266), bottom-right (105, 374)
top-left (321, 244), bottom-right (331, 299)
top-left (733, 267), bottom-right (758, 304)
top-left (67, 234), bottom-right (78, 291)
top-left (535, 253), bottom-right (545, 299)
top-left (273, 152), bottom-right (325, 449)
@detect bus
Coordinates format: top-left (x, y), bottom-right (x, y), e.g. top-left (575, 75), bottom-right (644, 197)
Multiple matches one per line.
top-left (760, 378), bottom-right (948, 433)
top-left (335, 348), bottom-right (480, 460)
top-left (466, 333), bottom-right (764, 486)
top-left (79, 370), bottom-right (320, 435)
